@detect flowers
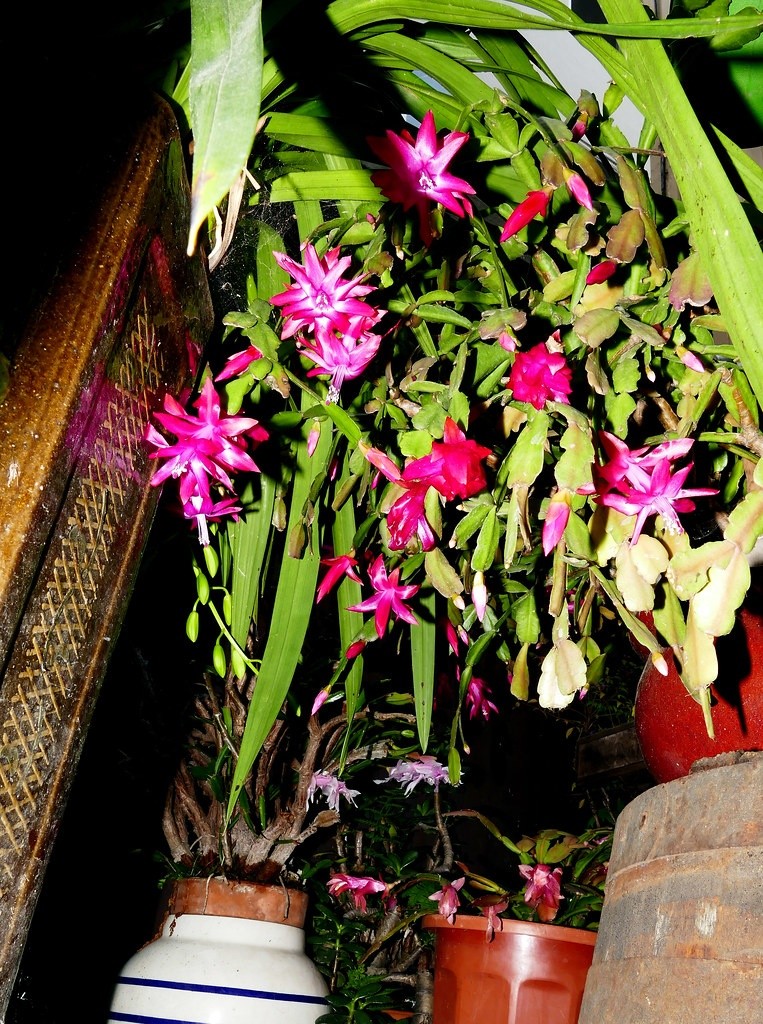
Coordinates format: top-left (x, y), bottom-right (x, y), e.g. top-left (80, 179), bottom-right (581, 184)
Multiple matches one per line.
top-left (140, 2), bottom-right (763, 1024)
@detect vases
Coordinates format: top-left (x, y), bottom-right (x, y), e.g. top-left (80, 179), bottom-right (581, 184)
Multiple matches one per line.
top-left (421, 913), bottom-right (600, 1024)
top-left (630, 588), bottom-right (763, 782)
top-left (109, 877), bottom-right (335, 1024)
top-left (572, 723), bottom-right (658, 816)
